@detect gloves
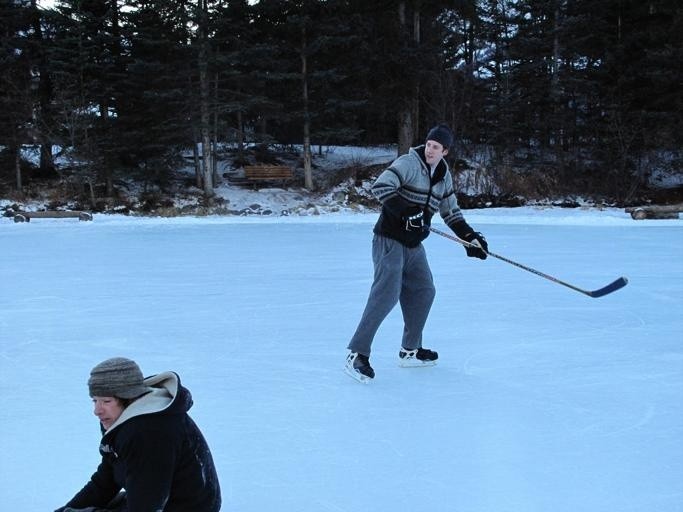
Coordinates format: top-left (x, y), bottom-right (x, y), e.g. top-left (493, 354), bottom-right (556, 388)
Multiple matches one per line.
top-left (400, 204), bottom-right (425, 233)
top-left (463, 231), bottom-right (487, 259)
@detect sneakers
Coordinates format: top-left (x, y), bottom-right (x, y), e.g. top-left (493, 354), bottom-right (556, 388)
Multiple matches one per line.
top-left (398, 347), bottom-right (437, 360)
top-left (346, 351), bottom-right (374, 379)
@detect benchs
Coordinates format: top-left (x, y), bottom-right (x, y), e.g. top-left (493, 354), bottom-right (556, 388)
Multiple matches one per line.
top-left (244, 164), bottom-right (294, 191)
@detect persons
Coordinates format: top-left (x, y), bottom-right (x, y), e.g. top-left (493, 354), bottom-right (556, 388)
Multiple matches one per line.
top-left (56, 355), bottom-right (222, 512)
top-left (345, 120), bottom-right (489, 380)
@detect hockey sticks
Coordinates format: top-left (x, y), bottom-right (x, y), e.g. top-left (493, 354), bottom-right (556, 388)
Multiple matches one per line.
top-left (429, 226), bottom-right (628, 297)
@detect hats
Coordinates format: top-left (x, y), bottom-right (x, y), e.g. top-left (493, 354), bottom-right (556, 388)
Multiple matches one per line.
top-left (87, 357), bottom-right (153, 400)
top-left (425, 123), bottom-right (453, 149)
